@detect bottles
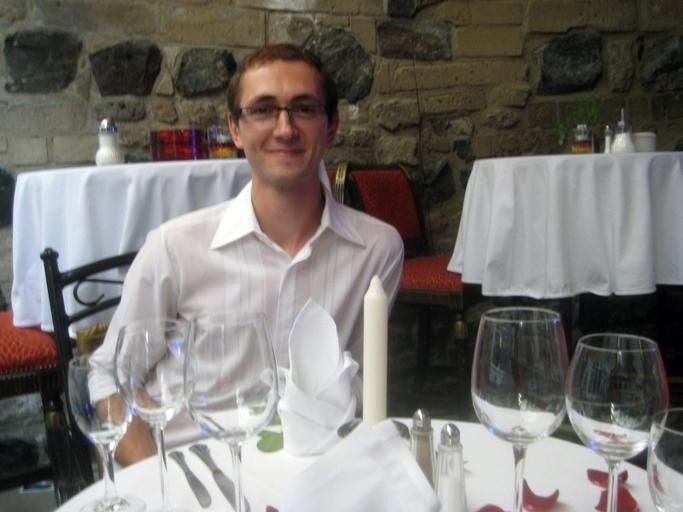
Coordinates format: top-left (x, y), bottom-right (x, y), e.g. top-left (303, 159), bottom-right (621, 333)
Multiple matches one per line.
top-left (433, 424), bottom-right (467, 512)
top-left (95, 115), bottom-right (125, 167)
top-left (409, 410), bottom-right (434, 485)
top-left (603, 125), bottom-right (612, 154)
top-left (611, 122), bottom-right (634, 154)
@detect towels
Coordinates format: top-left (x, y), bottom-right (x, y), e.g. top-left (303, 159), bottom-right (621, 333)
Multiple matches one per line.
top-left (283, 416), bottom-right (442, 512)
top-left (261, 296), bottom-right (360, 457)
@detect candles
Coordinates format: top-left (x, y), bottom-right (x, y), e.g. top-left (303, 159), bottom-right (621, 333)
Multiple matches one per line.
top-left (362, 273), bottom-right (389, 424)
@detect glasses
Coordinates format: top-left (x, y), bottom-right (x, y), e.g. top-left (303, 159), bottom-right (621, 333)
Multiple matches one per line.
top-left (235, 104), bottom-right (327, 120)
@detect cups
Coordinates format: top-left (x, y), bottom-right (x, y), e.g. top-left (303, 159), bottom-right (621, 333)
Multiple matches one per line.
top-left (207, 125), bottom-right (236, 160)
top-left (635, 131), bottom-right (656, 153)
top-left (572, 126), bottom-right (595, 155)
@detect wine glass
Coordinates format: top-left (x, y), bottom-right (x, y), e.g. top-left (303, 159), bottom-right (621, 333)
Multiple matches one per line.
top-left (646, 408), bottom-right (683, 512)
top-left (114, 318), bottom-right (197, 510)
top-left (183, 312), bottom-right (280, 511)
top-left (565, 333), bottom-right (668, 512)
top-left (471, 307), bottom-right (569, 511)
top-left (68, 359), bottom-right (146, 512)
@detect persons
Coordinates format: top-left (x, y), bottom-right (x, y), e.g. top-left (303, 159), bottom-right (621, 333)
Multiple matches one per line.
top-left (86, 44), bottom-right (404, 483)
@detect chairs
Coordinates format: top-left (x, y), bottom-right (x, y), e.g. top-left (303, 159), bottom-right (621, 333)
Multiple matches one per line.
top-left (38, 246), bottom-right (140, 492)
top-left (346, 162), bottom-right (480, 422)
top-left (323, 158), bottom-right (352, 205)
top-left (1, 307), bottom-right (80, 507)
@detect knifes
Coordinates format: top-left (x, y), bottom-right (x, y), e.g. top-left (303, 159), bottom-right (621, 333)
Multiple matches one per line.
top-left (192, 445), bottom-right (250, 511)
top-left (168, 450), bottom-right (211, 508)
